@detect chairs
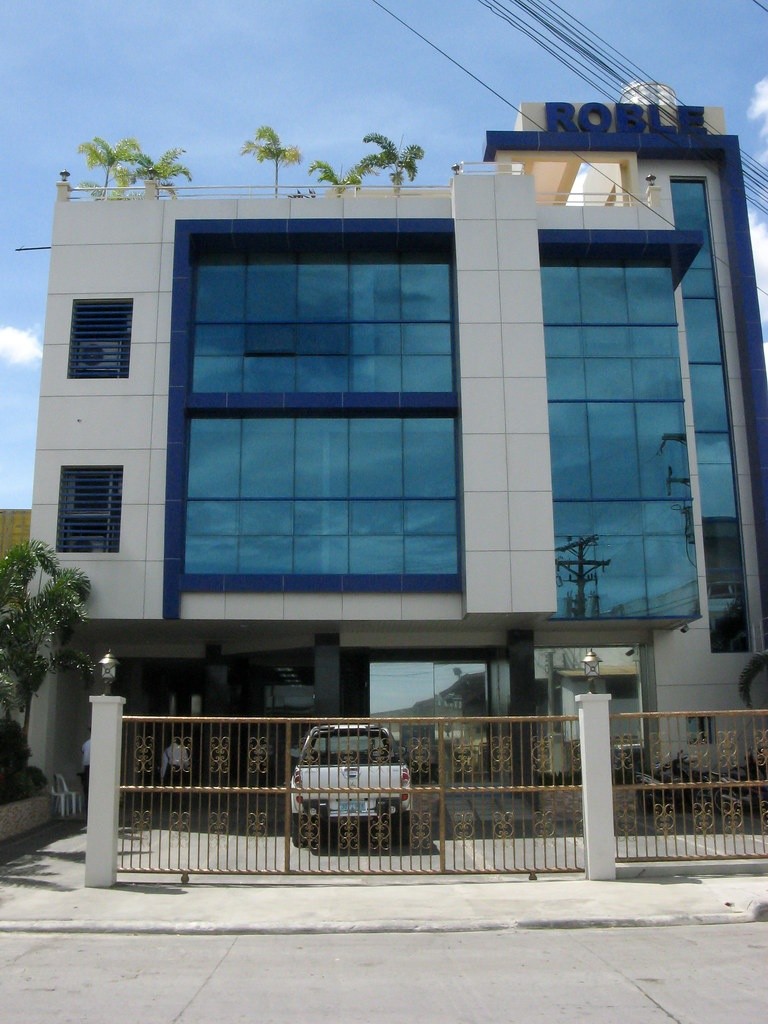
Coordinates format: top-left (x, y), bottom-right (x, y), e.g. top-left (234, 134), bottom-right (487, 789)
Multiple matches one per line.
top-left (51, 775), bottom-right (71, 817)
top-left (56, 774), bottom-right (81, 816)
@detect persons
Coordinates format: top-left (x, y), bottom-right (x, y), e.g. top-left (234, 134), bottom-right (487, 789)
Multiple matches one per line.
top-left (158, 733), bottom-right (190, 819)
top-left (80, 726), bottom-right (92, 825)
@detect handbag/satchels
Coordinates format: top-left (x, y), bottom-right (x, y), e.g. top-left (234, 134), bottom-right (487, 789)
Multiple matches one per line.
top-left (166, 763), bottom-right (183, 778)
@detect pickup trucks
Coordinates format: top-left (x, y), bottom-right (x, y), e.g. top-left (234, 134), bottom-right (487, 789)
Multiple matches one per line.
top-left (291, 724), bottom-right (412, 848)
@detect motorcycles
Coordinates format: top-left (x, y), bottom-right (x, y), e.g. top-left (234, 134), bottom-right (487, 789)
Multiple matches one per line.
top-left (635, 749), bottom-right (712, 816)
top-left (709, 748), bottom-right (768, 816)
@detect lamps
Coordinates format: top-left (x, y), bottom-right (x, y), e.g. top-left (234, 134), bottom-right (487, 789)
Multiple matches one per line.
top-left (148, 167), bottom-right (156, 180)
top-left (581, 648), bottom-right (603, 695)
top-left (60, 169), bottom-right (70, 181)
top-left (98, 648), bottom-right (121, 696)
top-left (646, 174), bottom-right (656, 186)
top-left (452, 163), bottom-right (460, 175)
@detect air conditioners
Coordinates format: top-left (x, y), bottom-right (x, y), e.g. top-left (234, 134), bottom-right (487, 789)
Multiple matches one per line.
top-left (77, 338), bottom-right (119, 370)
top-left (68, 535), bottom-right (106, 553)
